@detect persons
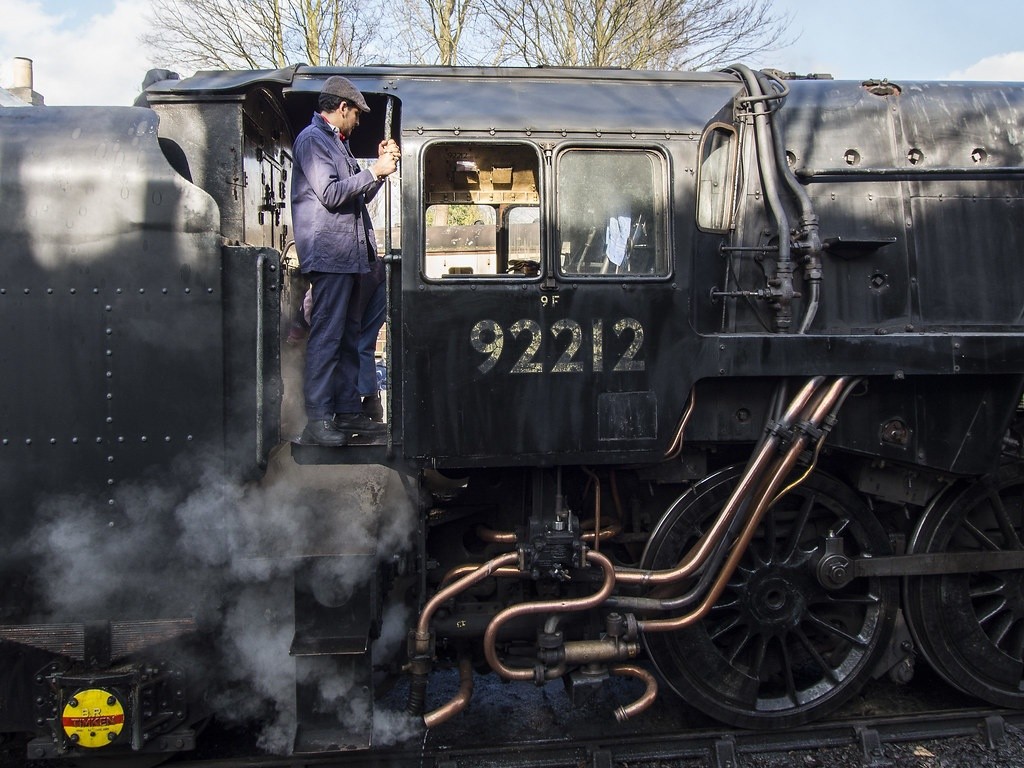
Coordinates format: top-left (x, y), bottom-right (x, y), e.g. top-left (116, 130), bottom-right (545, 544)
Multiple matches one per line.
top-left (290, 75), bottom-right (402, 448)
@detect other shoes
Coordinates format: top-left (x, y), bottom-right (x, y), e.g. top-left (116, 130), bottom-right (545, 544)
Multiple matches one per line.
top-left (362, 395), bottom-right (384, 423)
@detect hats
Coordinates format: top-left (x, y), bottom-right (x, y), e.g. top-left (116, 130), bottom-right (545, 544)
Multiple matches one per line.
top-left (321, 76), bottom-right (370, 113)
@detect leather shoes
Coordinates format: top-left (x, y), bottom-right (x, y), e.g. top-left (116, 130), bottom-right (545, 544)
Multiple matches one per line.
top-left (333, 414), bottom-right (387, 436)
top-left (301, 419), bottom-right (346, 447)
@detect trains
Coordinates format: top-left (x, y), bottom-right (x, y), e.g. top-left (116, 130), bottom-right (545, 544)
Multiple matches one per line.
top-left (0, 62), bottom-right (1023, 768)
